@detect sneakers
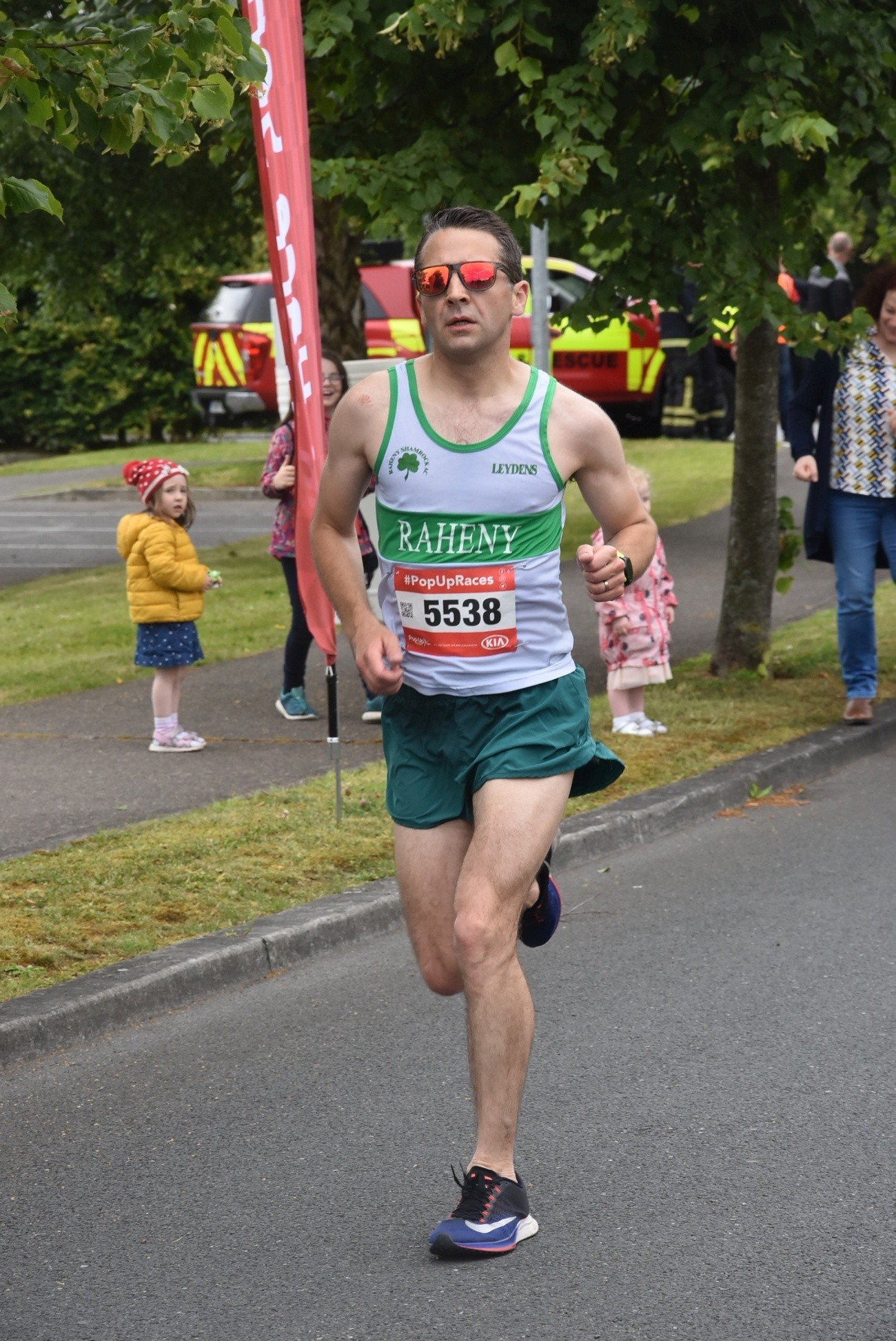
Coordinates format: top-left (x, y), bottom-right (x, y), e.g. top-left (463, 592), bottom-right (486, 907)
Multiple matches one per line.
top-left (362, 695), bottom-right (382, 721)
top-left (429, 1160), bottom-right (539, 1254)
top-left (274, 686), bottom-right (319, 720)
top-left (517, 828), bottom-right (562, 948)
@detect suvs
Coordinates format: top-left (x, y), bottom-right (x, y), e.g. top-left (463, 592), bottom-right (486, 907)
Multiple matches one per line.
top-left (191, 239), bottom-right (746, 440)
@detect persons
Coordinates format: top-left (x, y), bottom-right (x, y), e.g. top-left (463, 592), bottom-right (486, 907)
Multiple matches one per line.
top-left (661, 229), bottom-right (859, 448)
top-left (786, 262), bottom-right (896, 724)
top-left (590, 463), bottom-right (679, 738)
top-left (259, 349), bottom-right (385, 723)
top-left (307, 207), bottom-right (659, 1259)
top-left (115, 458), bottom-right (223, 753)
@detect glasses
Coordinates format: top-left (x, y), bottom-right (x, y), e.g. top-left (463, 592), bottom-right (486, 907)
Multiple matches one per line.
top-left (321, 374), bottom-right (345, 386)
top-left (412, 261), bottom-right (518, 297)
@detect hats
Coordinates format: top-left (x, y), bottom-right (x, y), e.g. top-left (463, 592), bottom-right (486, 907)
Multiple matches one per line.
top-left (122, 459), bottom-right (190, 506)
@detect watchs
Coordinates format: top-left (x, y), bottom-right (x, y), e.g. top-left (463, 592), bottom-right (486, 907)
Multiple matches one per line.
top-left (615, 551), bottom-right (633, 590)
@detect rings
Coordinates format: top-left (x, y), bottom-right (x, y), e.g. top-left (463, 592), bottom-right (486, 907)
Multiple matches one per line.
top-left (604, 580), bottom-right (609, 592)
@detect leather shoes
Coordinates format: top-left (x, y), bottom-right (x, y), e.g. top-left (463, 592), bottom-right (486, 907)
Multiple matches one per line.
top-left (842, 699), bottom-right (873, 725)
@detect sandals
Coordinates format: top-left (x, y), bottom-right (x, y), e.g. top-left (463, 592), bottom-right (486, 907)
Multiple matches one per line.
top-left (175, 727), bottom-right (207, 746)
top-left (635, 718), bottom-right (668, 734)
top-left (611, 719), bottom-right (654, 736)
top-left (148, 731), bottom-right (204, 753)
